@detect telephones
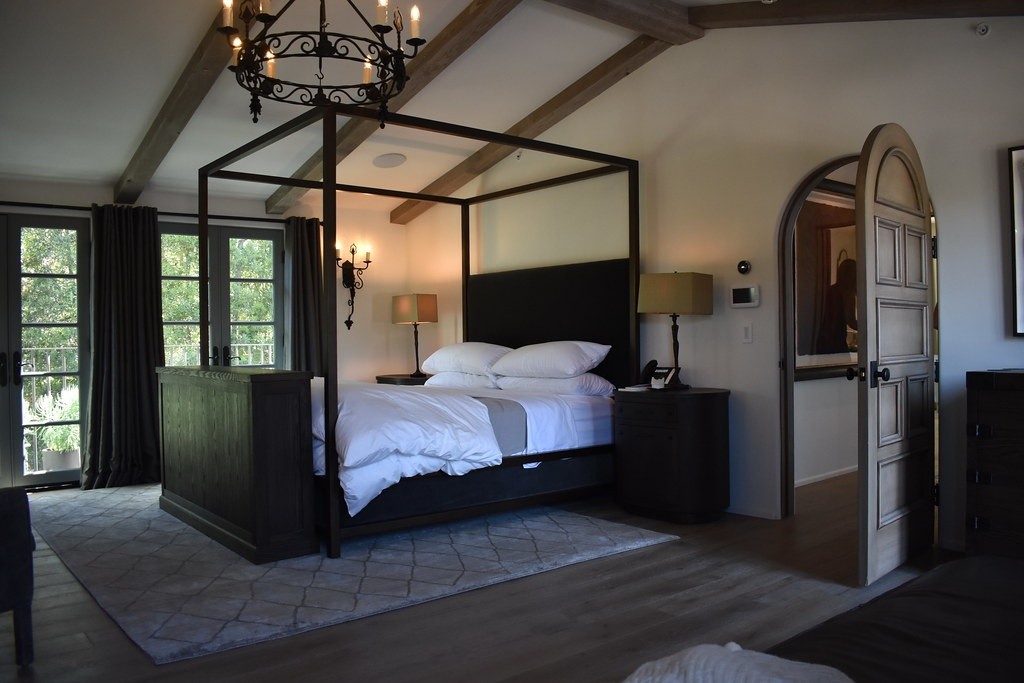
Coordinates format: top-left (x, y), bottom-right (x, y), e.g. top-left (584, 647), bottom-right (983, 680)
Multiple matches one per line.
top-left (638, 359), bottom-right (681, 385)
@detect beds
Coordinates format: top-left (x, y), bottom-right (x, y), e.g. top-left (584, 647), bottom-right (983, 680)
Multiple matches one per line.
top-left (622, 556), bottom-right (1024, 683)
top-left (198, 101), bottom-right (641, 561)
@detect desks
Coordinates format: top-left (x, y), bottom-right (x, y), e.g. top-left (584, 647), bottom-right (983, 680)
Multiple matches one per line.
top-left (0, 486), bottom-right (36, 668)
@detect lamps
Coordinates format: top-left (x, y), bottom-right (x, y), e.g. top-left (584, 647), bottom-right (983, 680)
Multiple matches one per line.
top-left (390, 293), bottom-right (438, 379)
top-left (336, 243), bottom-right (373, 330)
top-left (637, 270), bottom-right (713, 390)
top-left (219, 0), bottom-right (427, 130)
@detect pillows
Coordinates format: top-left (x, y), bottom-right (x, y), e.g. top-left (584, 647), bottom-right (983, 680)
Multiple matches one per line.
top-left (421, 341), bottom-right (514, 378)
top-left (423, 371), bottom-right (497, 388)
top-left (496, 372), bottom-right (617, 396)
top-left (490, 341), bottom-right (611, 378)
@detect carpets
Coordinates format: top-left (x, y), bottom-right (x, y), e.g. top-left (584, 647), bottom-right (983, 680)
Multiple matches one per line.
top-left (27, 482), bottom-right (682, 667)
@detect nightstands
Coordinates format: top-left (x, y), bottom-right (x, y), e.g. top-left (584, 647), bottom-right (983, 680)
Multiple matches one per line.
top-left (612, 386), bottom-right (731, 524)
top-left (377, 374), bottom-right (433, 386)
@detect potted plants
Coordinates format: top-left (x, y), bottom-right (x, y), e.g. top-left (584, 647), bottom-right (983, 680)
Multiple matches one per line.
top-left (30, 385), bottom-right (81, 471)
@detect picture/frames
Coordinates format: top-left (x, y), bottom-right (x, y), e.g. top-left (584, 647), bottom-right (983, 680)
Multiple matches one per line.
top-left (1008, 146), bottom-right (1024, 338)
top-left (792, 178), bottom-right (858, 381)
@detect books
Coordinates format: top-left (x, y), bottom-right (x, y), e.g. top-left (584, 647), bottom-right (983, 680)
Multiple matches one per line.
top-left (618, 367), bottom-right (676, 393)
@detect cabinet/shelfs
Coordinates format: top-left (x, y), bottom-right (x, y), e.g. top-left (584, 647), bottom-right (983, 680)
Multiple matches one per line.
top-left (154, 366), bottom-right (321, 566)
top-left (965, 368), bottom-right (1024, 559)
